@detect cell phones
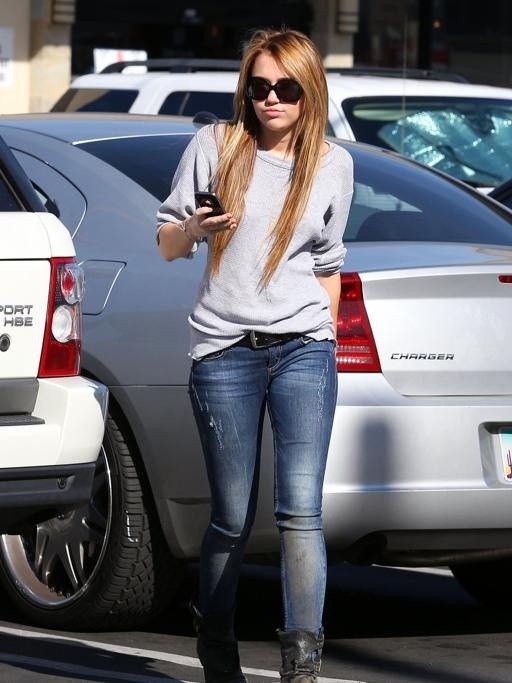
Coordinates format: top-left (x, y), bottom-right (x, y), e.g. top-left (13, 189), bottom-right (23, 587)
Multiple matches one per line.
top-left (195, 192), bottom-right (226, 218)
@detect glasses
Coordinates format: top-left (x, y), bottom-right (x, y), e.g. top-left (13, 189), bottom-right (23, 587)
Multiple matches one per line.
top-left (244, 76), bottom-right (303, 102)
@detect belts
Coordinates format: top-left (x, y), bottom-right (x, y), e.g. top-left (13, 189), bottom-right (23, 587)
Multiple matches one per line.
top-left (233, 330), bottom-right (300, 348)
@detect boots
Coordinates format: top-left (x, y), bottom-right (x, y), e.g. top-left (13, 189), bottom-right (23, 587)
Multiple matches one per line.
top-left (189, 597), bottom-right (248, 683)
top-left (274, 626), bottom-right (325, 683)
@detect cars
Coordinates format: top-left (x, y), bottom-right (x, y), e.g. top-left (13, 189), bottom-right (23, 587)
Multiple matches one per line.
top-left (0, 110), bottom-right (512, 630)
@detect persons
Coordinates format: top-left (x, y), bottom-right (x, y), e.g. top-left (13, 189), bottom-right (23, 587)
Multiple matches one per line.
top-left (155, 31), bottom-right (354, 683)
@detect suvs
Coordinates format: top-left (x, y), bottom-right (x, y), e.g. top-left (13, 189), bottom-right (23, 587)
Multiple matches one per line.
top-left (45, 58), bottom-right (512, 214)
top-left (0, 127), bottom-right (111, 536)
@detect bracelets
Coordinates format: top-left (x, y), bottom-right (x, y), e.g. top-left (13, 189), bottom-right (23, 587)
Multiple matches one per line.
top-left (176, 220), bottom-right (196, 242)
